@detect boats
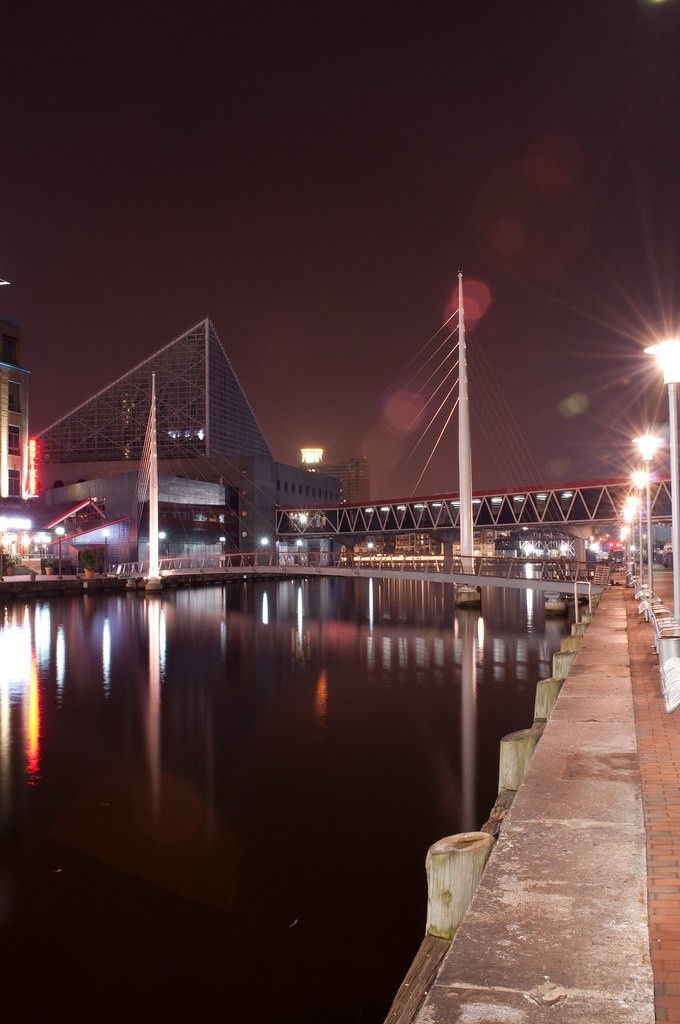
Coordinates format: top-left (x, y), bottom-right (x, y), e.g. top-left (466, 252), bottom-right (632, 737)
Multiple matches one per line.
top-left (453, 584), bottom-right (482, 607)
top-left (544, 597), bottom-right (569, 618)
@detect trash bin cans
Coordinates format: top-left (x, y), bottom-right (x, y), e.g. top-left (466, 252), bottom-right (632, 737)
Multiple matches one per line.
top-left (626, 562), bottom-right (636, 588)
top-left (657, 627), bottom-right (680, 668)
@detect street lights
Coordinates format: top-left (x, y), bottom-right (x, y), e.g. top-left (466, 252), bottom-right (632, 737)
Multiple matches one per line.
top-left (158, 532), bottom-right (167, 559)
top-left (296, 539), bottom-right (303, 566)
top-left (629, 471), bottom-right (650, 585)
top-left (104, 528), bottom-right (111, 576)
top-left (263, 539), bottom-right (268, 565)
top-left (219, 536), bottom-right (226, 565)
top-left (643, 334), bottom-right (680, 625)
top-left (630, 431), bottom-right (665, 601)
top-left (367, 544), bottom-right (373, 567)
top-left (55, 526), bottom-right (64, 580)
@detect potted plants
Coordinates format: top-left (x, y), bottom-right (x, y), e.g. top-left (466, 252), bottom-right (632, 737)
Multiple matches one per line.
top-left (80, 549), bottom-right (96, 578)
top-left (5, 560), bottom-right (16, 576)
top-left (44, 558), bottom-right (55, 575)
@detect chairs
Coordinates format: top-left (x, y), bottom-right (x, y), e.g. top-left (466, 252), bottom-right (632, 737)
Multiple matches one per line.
top-left (623, 572), bottom-right (680, 713)
